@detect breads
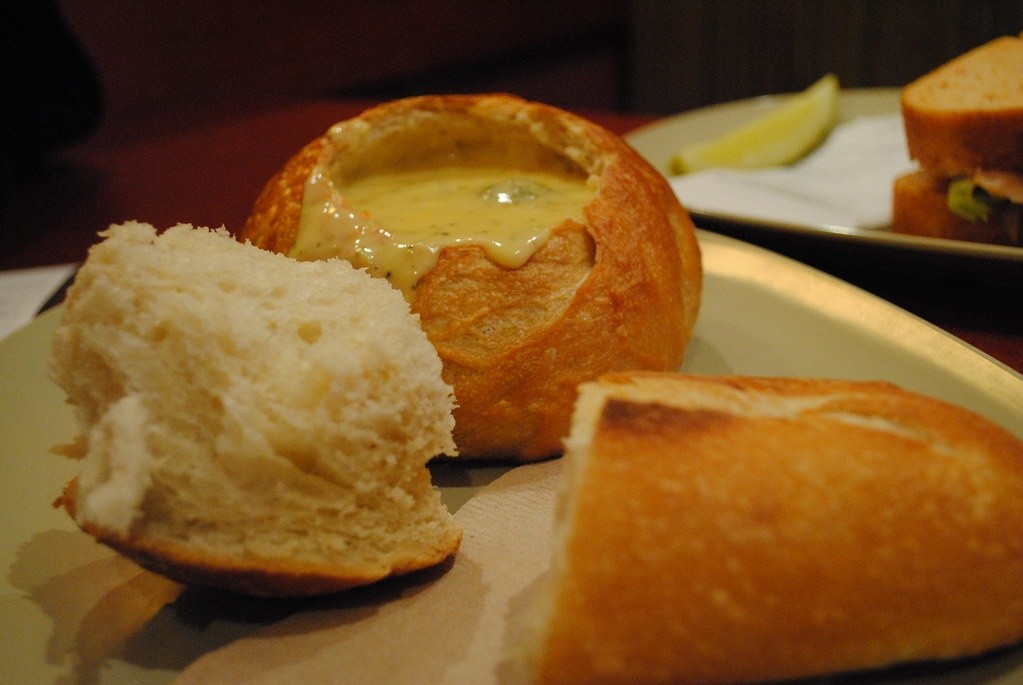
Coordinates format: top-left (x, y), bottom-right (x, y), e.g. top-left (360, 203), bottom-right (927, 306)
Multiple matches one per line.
top-left (892, 168), bottom-right (1023, 249)
top-left (494, 366), bottom-right (1023, 685)
top-left (46, 218), bottom-right (462, 600)
top-left (900, 30), bottom-right (1023, 166)
top-left (242, 92), bottom-right (704, 462)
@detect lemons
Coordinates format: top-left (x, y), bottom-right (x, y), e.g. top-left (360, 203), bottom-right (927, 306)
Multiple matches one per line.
top-left (669, 71), bottom-right (838, 173)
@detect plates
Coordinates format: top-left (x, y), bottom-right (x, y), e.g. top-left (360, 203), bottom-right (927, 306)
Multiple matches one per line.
top-left (619, 87), bottom-right (1023, 257)
top-left (0, 230), bottom-right (1021, 685)
